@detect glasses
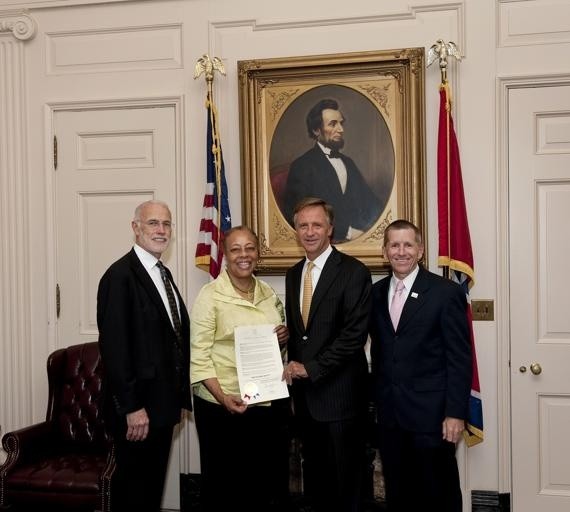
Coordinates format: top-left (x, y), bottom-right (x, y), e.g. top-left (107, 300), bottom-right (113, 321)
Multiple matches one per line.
top-left (136, 218), bottom-right (174, 231)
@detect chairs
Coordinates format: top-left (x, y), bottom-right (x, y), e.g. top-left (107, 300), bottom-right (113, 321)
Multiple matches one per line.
top-left (2, 341), bottom-right (119, 511)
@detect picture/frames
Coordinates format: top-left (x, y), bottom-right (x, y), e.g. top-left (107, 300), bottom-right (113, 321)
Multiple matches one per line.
top-left (237, 45), bottom-right (431, 274)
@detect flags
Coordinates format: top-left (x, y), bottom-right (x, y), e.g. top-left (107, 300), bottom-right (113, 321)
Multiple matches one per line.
top-left (437, 82), bottom-right (483, 447)
top-left (194, 94), bottom-right (232, 280)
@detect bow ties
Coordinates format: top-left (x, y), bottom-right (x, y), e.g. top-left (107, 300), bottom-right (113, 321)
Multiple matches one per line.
top-left (325, 151), bottom-right (341, 159)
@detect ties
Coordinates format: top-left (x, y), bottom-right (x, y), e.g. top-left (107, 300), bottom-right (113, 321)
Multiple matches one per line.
top-left (301, 261), bottom-right (316, 334)
top-left (389, 279), bottom-right (408, 333)
top-left (155, 259), bottom-right (184, 349)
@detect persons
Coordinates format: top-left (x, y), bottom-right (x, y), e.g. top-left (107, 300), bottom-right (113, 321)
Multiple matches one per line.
top-left (368, 220), bottom-right (473, 512)
top-left (285, 99), bottom-right (384, 242)
top-left (284, 197), bottom-right (374, 512)
top-left (97, 200), bottom-right (192, 512)
top-left (189, 225), bottom-right (291, 512)
